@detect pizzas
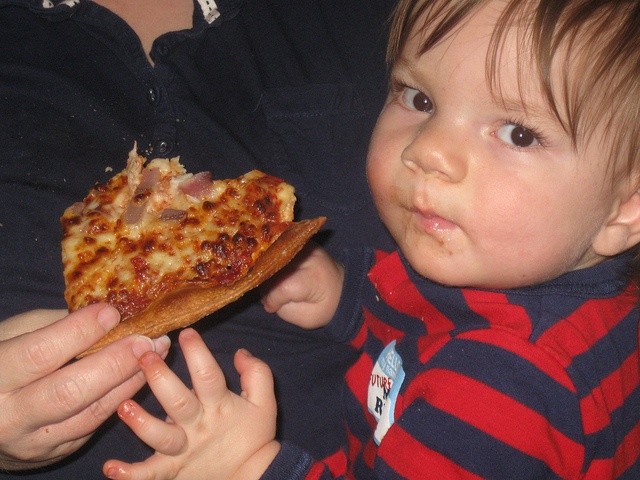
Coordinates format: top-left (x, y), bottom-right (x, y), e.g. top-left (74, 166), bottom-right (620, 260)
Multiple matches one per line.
top-left (58, 141), bottom-right (327, 359)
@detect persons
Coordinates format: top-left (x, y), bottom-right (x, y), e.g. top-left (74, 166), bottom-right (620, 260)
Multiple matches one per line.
top-left (102, 2), bottom-right (640, 480)
top-left (0, 0), bottom-right (422, 479)
